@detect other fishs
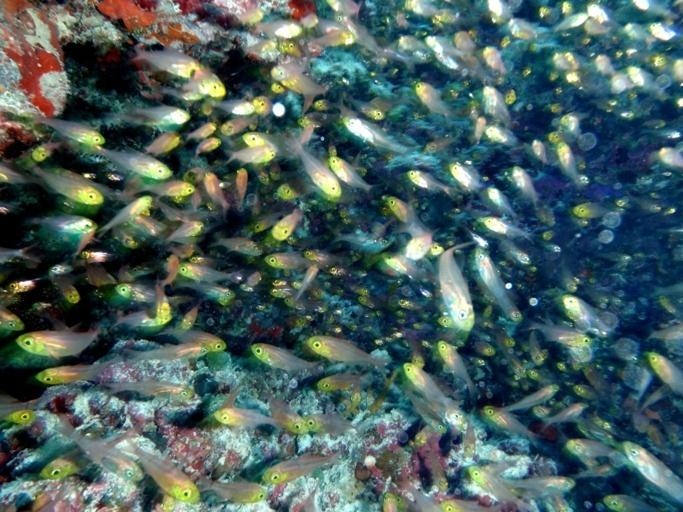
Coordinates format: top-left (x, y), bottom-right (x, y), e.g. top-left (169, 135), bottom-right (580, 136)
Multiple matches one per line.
top-left (0, 1), bottom-right (682, 512)
